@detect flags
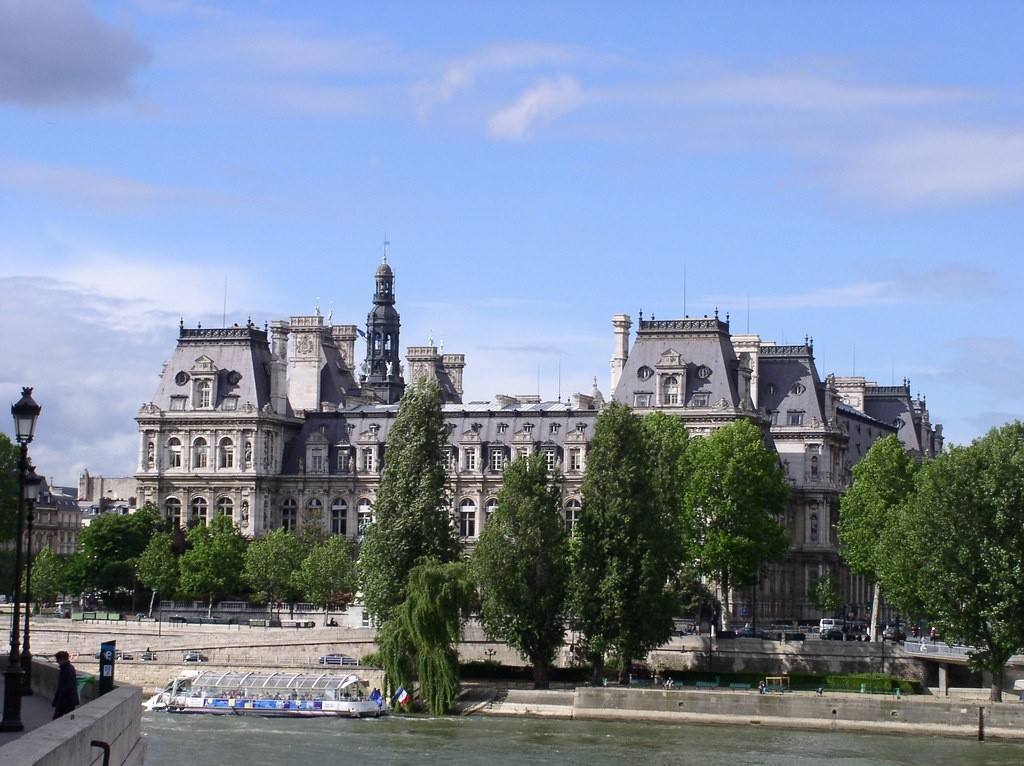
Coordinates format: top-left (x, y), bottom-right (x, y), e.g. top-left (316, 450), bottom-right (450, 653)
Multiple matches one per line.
top-left (368, 689), bottom-right (384, 709)
top-left (394, 686), bottom-right (411, 707)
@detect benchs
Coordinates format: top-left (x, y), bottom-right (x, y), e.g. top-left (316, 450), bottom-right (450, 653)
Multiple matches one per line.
top-left (627, 679), bottom-right (789, 693)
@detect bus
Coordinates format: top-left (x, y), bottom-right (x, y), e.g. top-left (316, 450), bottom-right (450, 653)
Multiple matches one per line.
top-left (0, 602), bottom-right (33, 614)
top-left (819, 618), bottom-right (868, 640)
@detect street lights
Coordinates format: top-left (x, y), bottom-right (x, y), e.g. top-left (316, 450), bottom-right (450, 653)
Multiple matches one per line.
top-left (20, 465), bottom-right (44, 696)
top-left (484, 648), bottom-right (497, 684)
top-left (0, 386), bottom-right (42, 733)
top-left (132, 564), bottom-right (137, 616)
top-left (868, 656), bottom-right (876, 694)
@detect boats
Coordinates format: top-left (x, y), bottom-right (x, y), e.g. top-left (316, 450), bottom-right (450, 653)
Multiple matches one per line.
top-left (152, 670), bottom-right (394, 718)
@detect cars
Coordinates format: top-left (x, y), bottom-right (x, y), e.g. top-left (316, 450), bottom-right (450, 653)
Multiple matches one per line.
top-left (94, 649), bottom-right (134, 660)
top-left (470, 657), bottom-right (486, 664)
top-left (930, 627), bottom-right (945, 642)
top-left (735, 627), bottom-right (768, 639)
top-left (183, 652), bottom-right (208, 663)
top-left (41, 602), bottom-right (74, 618)
top-left (140, 652), bottom-right (158, 660)
top-left (319, 653), bottom-right (362, 666)
top-left (882, 627), bottom-right (907, 642)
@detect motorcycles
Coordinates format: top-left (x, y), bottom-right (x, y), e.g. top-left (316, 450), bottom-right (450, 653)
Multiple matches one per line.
top-left (326, 621), bottom-right (339, 626)
top-left (911, 627), bottom-right (921, 639)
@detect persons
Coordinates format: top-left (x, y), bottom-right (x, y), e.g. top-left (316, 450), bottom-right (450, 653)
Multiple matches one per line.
top-left (51, 650), bottom-right (80, 719)
top-left (664, 675), bottom-right (823, 698)
top-left (190, 688), bottom-right (380, 701)
top-left (909, 623), bottom-right (938, 645)
